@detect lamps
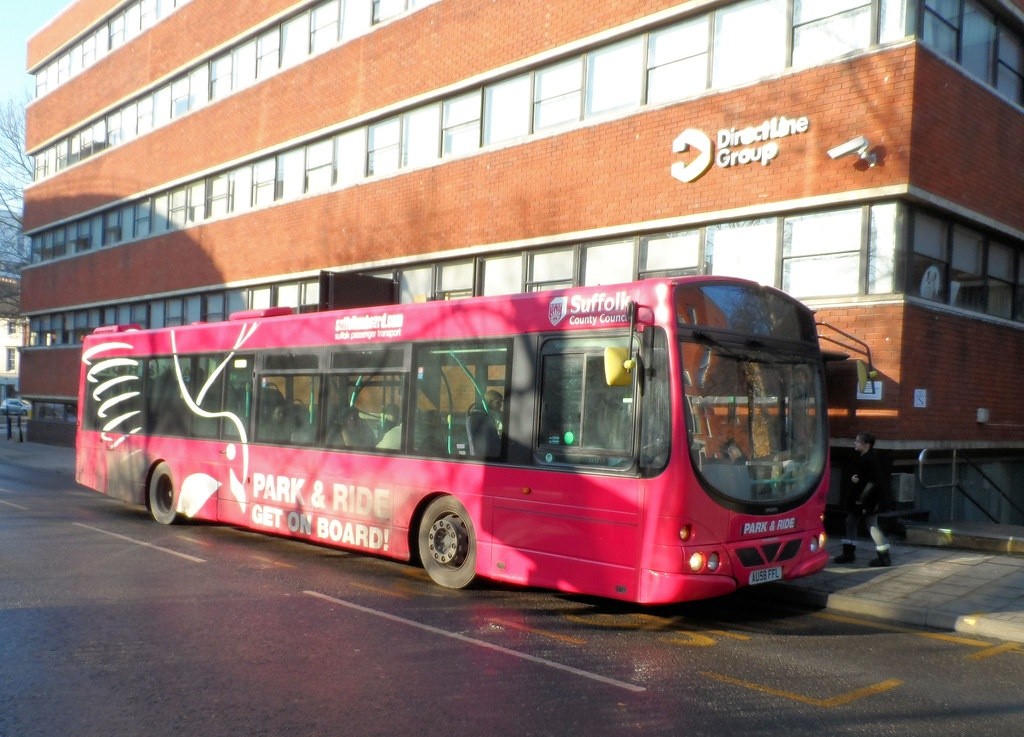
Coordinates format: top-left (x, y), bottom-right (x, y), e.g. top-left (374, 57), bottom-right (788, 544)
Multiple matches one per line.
top-left (976, 406), bottom-right (988, 424)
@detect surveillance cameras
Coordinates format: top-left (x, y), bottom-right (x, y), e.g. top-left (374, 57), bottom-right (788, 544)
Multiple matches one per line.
top-left (828, 135), bottom-right (875, 168)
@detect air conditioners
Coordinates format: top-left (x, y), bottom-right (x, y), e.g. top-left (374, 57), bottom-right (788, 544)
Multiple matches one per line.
top-left (891, 473), bottom-right (916, 503)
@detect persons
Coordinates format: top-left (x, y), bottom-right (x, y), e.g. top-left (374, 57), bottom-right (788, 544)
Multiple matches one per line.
top-left (834, 429), bottom-right (892, 566)
top-left (333, 406), bottom-right (376, 449)
top-left (259, 402), bottom-right (291, 440)
top-left (482, 389), bottom-right (503, 440)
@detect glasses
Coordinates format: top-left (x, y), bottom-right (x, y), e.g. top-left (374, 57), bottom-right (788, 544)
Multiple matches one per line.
top-left (855, 439), bottom-right (865, 444)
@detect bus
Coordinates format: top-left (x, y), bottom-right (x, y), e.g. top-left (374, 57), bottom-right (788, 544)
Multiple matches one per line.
top-left (73, 276), bottom-right (878, 607)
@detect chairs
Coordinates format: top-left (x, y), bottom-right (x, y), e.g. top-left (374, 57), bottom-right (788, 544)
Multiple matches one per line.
top-left (146, 365), bottom-right (502, 461)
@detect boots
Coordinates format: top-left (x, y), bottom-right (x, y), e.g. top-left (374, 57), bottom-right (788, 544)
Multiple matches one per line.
top-left (867, 547), bottom-right (893, 567)
top-left (833, 543), bottom-right (857, 564)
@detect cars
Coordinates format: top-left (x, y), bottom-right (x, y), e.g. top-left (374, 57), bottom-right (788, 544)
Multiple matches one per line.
top-left (0, 398), bottom-right (32, 416)
